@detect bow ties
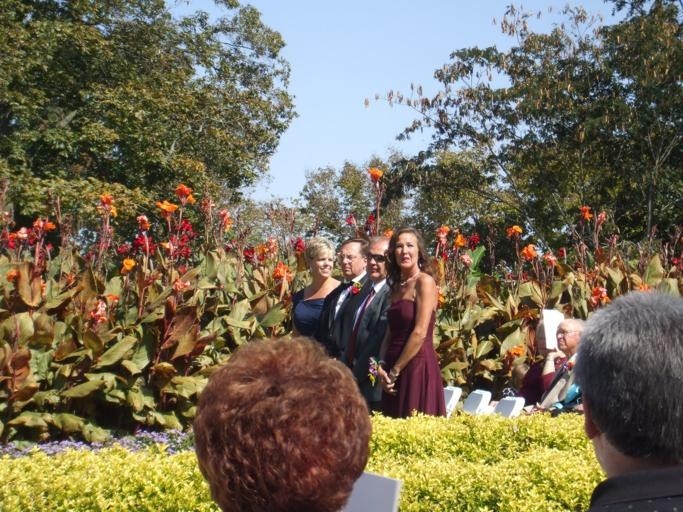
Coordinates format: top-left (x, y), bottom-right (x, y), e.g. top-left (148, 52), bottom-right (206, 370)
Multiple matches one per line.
top-left (342, 282), bottom-right (354, 289)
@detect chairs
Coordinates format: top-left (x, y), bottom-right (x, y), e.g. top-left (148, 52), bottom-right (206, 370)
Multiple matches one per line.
top-left (440, 384), bottom-right (526, 419)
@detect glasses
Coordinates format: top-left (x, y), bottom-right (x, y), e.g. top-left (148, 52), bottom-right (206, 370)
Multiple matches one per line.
top-left (365, 251), bottom-right (386, 262)
top-left (336, 253), bottom-right (358, 262)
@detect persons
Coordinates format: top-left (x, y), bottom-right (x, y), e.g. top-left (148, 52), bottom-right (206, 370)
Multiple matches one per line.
top-left (193, 334), bottom-right (372, 512)
top-left (572, 290), bottom-right (683, 512)
